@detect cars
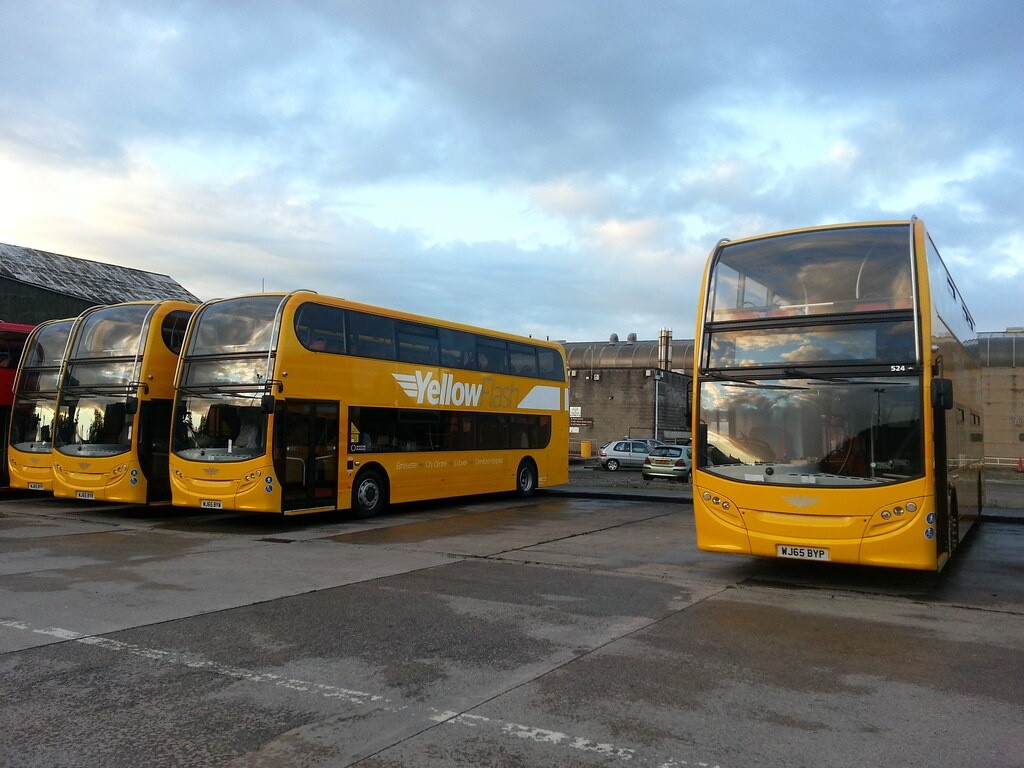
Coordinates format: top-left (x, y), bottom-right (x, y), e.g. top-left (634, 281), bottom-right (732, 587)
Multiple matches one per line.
top-left (598, 440), bottom-right (653, 472)
top-left (641, 445), bottom-right (713, 484)
top-left (626, 439), bottom-right (668, 452)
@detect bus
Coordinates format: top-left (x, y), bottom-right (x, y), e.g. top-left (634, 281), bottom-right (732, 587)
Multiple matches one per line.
top-left (167, 290), bottom-right (569, 521)
top-left (6, 297), bottom-right (534, 506)
top-left (686, 214), bottom-right (986, 572)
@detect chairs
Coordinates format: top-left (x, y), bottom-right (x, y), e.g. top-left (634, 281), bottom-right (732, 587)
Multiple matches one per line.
top-left (751, 426), bottom-right (788, 462)
top-left (302, 335), bottom-right (327, 352)
top-left (856, 291), bottom-right (913, 310)
top-left (830, 431), bottom-right (865, 474)
top-left (732, 297), bottom-right (796, 320)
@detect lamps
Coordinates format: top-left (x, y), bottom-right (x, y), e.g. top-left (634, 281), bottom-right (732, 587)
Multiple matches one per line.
top-left (608, 395), bottom-right (613, 400)
